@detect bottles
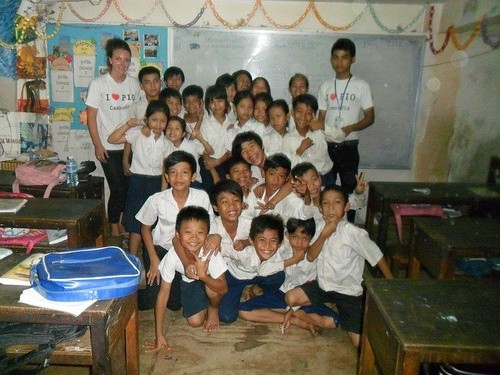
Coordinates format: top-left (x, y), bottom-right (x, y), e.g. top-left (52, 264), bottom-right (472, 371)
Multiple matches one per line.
top-left (66, 156), bottom-right (79, 187)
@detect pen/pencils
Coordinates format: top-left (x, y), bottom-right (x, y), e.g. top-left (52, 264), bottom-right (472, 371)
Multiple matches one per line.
top-left (282, 326), bottom-right (283, 334)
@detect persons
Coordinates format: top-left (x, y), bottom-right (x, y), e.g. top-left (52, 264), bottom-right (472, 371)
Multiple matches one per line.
top-left (107, 67), bottom-right (395, 352)
top-left (84, 38), bottom-right (151, 237)
top-left (317, 38), bottom-right (376, 224)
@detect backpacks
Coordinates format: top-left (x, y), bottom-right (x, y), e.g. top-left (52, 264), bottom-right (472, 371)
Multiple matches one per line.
top-left (29, 245), bottom-right (142, 302)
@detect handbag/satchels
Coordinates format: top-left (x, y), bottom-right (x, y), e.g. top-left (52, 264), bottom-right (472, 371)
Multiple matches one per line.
top-left (12, 159), bottom-right (67, 200)
top-left (0, 321), bottom-right (88, 375)
top-left (390, 205), bottom-right (443, 246)
top-left (457, 256), bottom-right (500, 283)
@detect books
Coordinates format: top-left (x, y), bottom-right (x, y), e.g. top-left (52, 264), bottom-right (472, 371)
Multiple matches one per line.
top-left (46, 229), bottom-right (69, 245)
top-left (0, 198), bottom-right (29, 213)
top-left (0, 248), bottom-right (98, 318)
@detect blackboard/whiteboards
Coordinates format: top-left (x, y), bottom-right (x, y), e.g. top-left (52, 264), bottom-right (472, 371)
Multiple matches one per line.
top-left (166, 26), bottom-right (426, 170)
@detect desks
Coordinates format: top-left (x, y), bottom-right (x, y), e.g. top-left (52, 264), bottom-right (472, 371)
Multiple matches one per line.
top-left (355, 278), bottom-right (500, 375)
top-left (0, 197), bottom-right (105, 250)
top-left (0, 170), bottom-right (104, 201)
top-left (410, 216), bottom-right (500, 279)
top-left (0, 253), bottom-right (143, 375)
top-left (364, 179), bottom-right (500, 251)
